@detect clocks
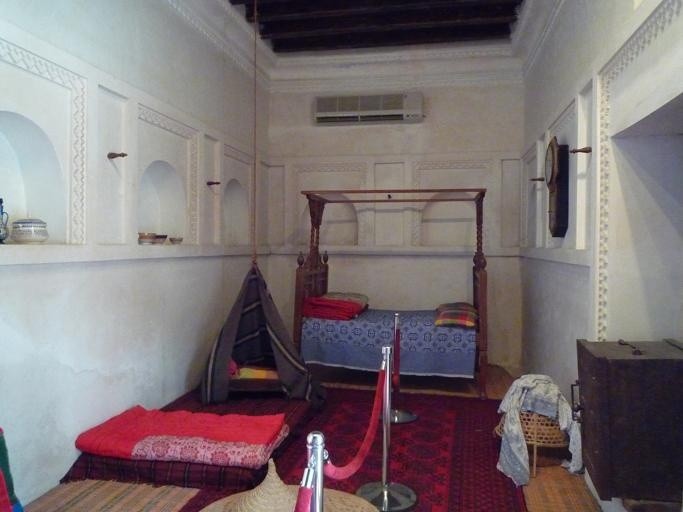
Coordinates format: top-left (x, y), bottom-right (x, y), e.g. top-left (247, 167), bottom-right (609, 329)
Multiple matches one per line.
top-left (545, 137), bottom-right (572, 238)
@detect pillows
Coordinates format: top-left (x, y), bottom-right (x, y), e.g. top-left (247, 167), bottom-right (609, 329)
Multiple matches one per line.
top-left (432, 310), bottom-right (476, 330)
top-left (432, 301), bottom-right (477, 317)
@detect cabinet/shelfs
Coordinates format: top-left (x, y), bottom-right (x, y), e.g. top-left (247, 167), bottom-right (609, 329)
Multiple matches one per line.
top-left (575, 331), bottom-right (683, 503)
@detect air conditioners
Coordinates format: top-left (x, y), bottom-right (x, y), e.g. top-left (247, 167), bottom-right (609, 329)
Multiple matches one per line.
top-left (313, 87), bottom-right (427, 124)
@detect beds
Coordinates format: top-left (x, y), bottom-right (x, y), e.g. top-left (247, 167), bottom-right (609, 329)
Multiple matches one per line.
top-left (293, 184), bottom-right (492, 401)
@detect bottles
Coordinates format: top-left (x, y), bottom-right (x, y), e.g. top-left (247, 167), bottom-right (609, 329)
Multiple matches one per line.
top-left (10, 212), bottom-right (47, 244)
top-left (0, 198), bottom-right (9, 242)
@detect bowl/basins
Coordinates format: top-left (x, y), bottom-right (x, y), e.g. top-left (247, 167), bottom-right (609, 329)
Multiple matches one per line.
top-left (139, 232), bottom-right (184, 245)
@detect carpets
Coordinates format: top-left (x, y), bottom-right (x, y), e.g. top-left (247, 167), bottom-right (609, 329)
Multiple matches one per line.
top-left (273, 391), bottom-right (527, 511)
